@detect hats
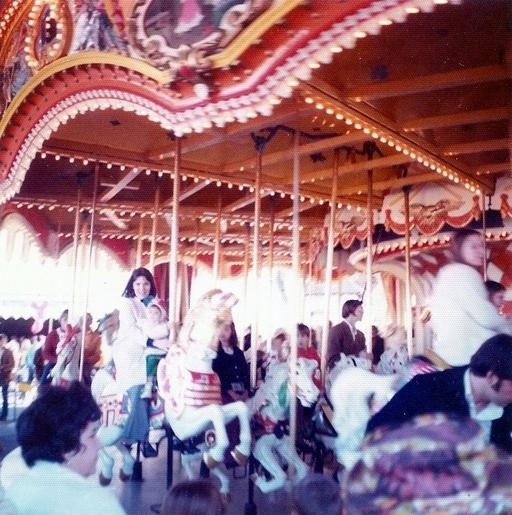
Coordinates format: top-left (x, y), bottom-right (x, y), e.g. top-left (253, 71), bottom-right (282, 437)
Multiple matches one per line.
top-left (147, 298), bottom-right (167, 320)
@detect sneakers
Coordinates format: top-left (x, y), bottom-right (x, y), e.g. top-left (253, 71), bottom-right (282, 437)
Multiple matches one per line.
top-left (141, 387), bottom-right (152, 398)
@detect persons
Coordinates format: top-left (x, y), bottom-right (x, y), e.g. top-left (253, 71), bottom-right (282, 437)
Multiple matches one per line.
top-left (134, 301), bottom-right (171, 400)
top-left (429, 228), bottom-right (512, 369)
top-left (116, 267), bottom-right (175, 447)
top-left (359, 335), bottom-right (512, 454)
top-left (1, 380), bottom-right (126, 514)
top-left (1, 280), bottom-right (511, 515)
